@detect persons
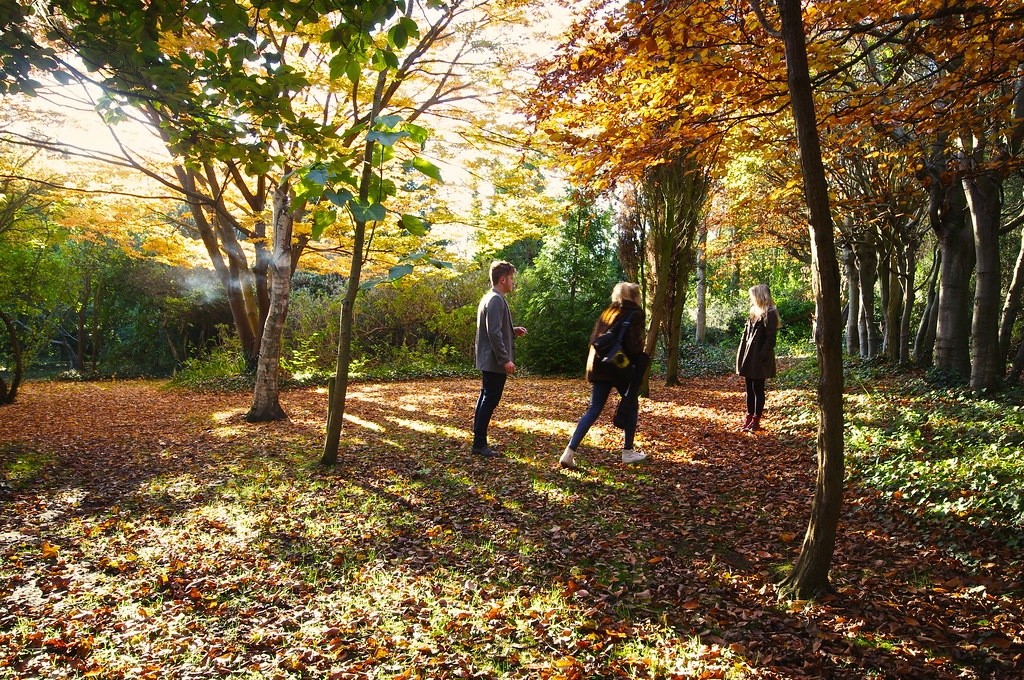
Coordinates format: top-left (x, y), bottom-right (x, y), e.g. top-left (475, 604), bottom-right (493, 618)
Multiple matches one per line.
top-left (471, 261), bottom-right (527, 455)
top-left (559, 282), bottom-right (647, 467)
top-left (735, 284), bottom-right (778, 433)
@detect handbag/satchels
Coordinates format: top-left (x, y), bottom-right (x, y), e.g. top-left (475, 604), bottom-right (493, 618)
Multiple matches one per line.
top-left (591, 308), bottom-right (643, 382)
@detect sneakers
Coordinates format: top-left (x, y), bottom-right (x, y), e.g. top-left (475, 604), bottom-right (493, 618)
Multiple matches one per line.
top-left (621, 448), bottom-right (648, 463)
top-left (559, 447), bottom-right (578, 469)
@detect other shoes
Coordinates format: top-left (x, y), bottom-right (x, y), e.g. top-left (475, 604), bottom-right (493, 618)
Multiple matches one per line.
top-left (742, 413), bottom-right (753, 428)
top-left (471, 445), bottom-right (501, 456)
top-left (741, 416), bottom-right (760, 432)
